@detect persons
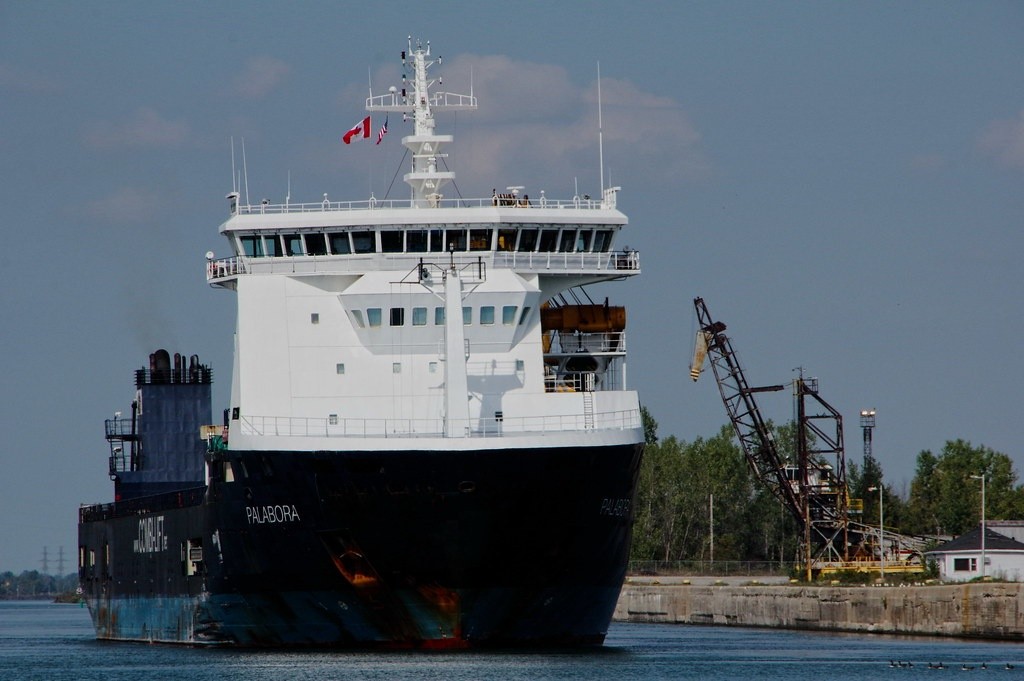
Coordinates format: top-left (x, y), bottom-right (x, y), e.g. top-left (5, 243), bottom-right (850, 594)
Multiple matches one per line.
top-left (221, 426), bottom-right (229, 445)
top-left (522, 195), bottom-right (529, 207)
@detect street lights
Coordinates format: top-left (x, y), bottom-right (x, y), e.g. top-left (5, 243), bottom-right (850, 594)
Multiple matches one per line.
top-left (970, 474), bottom-right (985, 577)
top-left (869, 485), bottom-right (884, 584)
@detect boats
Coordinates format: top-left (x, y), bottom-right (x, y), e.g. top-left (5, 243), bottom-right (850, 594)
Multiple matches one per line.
top-left (78, 36), bottom-right (647, 654)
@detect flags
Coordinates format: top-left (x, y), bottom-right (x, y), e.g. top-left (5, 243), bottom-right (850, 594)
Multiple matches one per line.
top-left (376, 115), bottom-right (388, 145)
top-left (340, 116), bottom-right (373, 145)
